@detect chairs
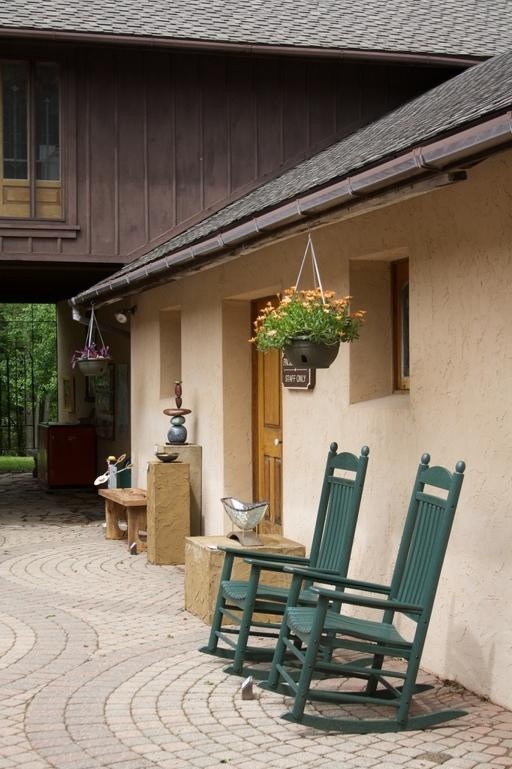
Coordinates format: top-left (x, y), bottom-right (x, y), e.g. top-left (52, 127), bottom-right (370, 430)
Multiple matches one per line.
top-left (267, 452), bottom-right (465, 728)
top-left (199, 443), bottom-right (370, 675)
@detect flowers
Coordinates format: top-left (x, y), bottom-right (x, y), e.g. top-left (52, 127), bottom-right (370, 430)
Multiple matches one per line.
top-left (73, 342), bottom-right (113, 364)
top-left (250, 286), bottom-right (368, 351)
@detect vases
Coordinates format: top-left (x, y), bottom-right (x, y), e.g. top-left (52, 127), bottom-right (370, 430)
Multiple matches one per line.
top-left (78, 358), bottom-right (111, 377)
top-left (280, 340), bottom-right (339, 370)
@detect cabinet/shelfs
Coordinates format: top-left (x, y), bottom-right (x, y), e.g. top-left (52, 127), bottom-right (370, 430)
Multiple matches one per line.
top-left (37, 421), bottom-right (111, 495)
top-left (97, 443), bottom-right (310, 627)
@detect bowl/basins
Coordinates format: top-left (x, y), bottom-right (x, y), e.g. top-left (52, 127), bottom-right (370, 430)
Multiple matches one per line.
top-left (155, 453), bottom-right (178, 462)
